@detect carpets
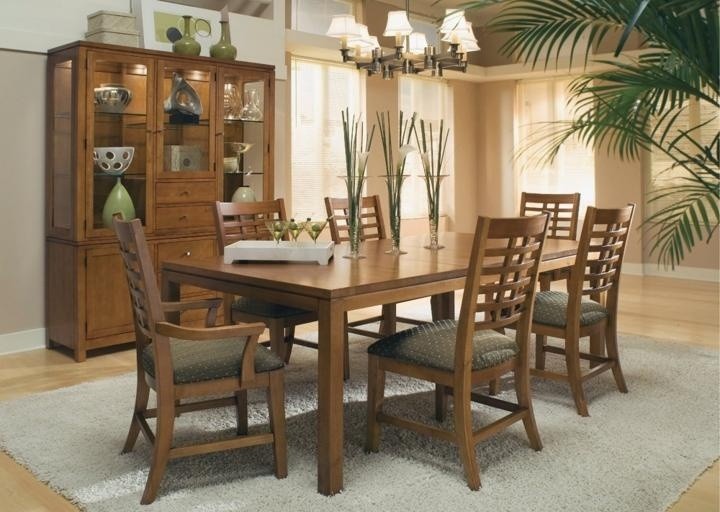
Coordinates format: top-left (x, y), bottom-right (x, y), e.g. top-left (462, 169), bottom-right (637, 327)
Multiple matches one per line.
top-left (0, 320), bottom-right (720, 512)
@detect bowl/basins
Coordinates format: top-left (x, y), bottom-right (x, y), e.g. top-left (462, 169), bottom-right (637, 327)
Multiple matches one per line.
top-left (224, 157), bottom-right (237, 174)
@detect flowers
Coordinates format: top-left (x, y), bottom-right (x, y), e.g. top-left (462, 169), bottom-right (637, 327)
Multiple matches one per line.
top-left (340, 105), bottom-right (452, 252)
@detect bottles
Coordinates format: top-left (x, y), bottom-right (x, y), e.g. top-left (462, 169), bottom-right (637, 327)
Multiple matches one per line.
top-left (240, 88), bottom-right (263, 121)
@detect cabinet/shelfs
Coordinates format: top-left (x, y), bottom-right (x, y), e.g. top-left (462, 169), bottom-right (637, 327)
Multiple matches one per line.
top-left (42, 39), bottom-right (277, 364)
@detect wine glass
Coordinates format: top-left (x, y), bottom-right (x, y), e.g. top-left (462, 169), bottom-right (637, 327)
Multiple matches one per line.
top-left (265, 219), bottom-right (328, 247)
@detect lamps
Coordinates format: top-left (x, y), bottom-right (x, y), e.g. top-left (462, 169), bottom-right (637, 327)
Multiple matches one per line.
top-left (325, 0), bottom-right (482, 81)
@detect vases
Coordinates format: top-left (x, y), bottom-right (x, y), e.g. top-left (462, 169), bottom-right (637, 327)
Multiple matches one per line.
top-left (172, 14), bottom-right (238, 62)
top-left (336, 174), bottom-right (450, 260)
